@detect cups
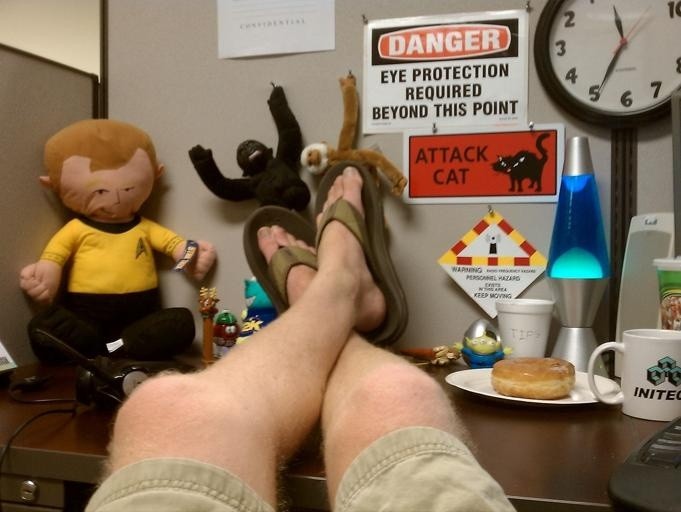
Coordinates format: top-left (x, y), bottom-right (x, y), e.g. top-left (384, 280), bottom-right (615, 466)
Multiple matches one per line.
top-left (653, 258), bottom-right (681, 331)
top-left (587, 327), bottom-right (681, 423)
top-left (494, 298), bottom-right (556, 358)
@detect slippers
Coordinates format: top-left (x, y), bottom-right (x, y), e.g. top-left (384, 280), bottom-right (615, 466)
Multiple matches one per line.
top-left (243, 205), bottom-right (317, 317)
top-left (315, 159), bottom-right (409, 347)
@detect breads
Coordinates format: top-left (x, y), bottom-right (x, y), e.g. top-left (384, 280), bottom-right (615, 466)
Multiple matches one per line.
top-left (491, 356), bottom-right (576, 400)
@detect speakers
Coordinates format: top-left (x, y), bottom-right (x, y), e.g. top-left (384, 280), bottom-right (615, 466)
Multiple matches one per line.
top-left (614, 212), bottom-right (676, 378)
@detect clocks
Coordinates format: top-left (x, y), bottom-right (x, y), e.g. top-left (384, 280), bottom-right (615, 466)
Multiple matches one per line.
top-left (533, 1), bottom-right (681, 127)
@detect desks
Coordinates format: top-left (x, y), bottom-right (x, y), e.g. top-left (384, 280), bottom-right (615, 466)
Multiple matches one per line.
top-left (0, 338), bottom-right (680, 511)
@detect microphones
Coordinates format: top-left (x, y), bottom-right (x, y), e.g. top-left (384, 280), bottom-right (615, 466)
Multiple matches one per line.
top-left (31, 329), bottom-right (113, 384)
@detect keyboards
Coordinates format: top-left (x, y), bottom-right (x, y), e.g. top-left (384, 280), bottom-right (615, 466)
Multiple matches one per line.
top-left (607, 416), bottom-right (681, 512)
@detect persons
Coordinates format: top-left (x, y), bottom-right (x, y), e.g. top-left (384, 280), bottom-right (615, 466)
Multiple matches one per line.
top-left (16, 117), bottom-right (218, 360)
top-left (78, 163), bottom-right (521, 511)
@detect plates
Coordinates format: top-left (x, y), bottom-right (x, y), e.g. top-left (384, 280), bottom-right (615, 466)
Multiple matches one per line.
top-left (446, 368), bottom-right (619, 407)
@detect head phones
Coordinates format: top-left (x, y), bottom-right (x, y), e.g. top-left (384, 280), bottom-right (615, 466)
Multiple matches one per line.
top-left (75, 355), bottom-right (198, 411)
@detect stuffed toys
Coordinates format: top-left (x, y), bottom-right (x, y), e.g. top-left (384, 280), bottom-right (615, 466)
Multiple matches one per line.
top-left (180, 81), bottom-right (308, 215)
top-left (297, 67), bottom-right (409, 193)
